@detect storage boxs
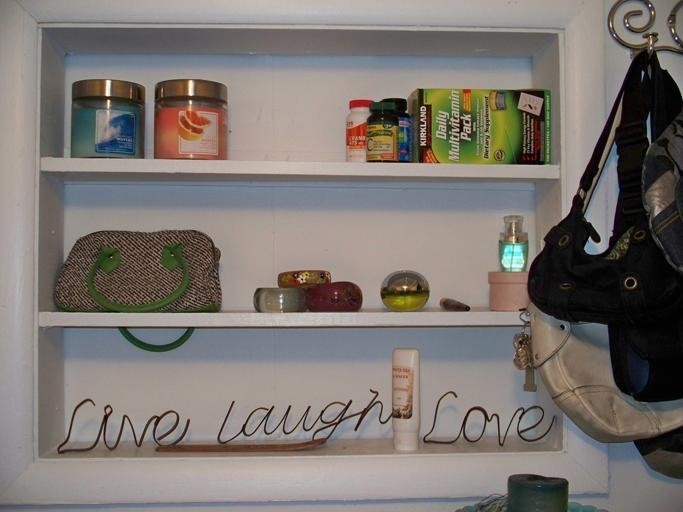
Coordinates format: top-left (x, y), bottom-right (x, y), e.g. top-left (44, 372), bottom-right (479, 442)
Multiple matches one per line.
top-left (407, 88), bottom-right (551, 166)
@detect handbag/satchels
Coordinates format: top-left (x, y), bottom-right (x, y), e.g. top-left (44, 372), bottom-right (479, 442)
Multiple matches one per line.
top-left (527, 47), bottom-right (683, 478)
top-left (53, 229), bottom-right (222, 313)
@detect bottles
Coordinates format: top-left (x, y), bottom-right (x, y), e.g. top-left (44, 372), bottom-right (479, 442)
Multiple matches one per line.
top-left (345, 98), bottom-right (413, 163)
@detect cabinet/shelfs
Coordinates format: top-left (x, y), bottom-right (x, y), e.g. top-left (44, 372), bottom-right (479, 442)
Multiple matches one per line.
top-left (34, 22), bottom-right (568, 463)
